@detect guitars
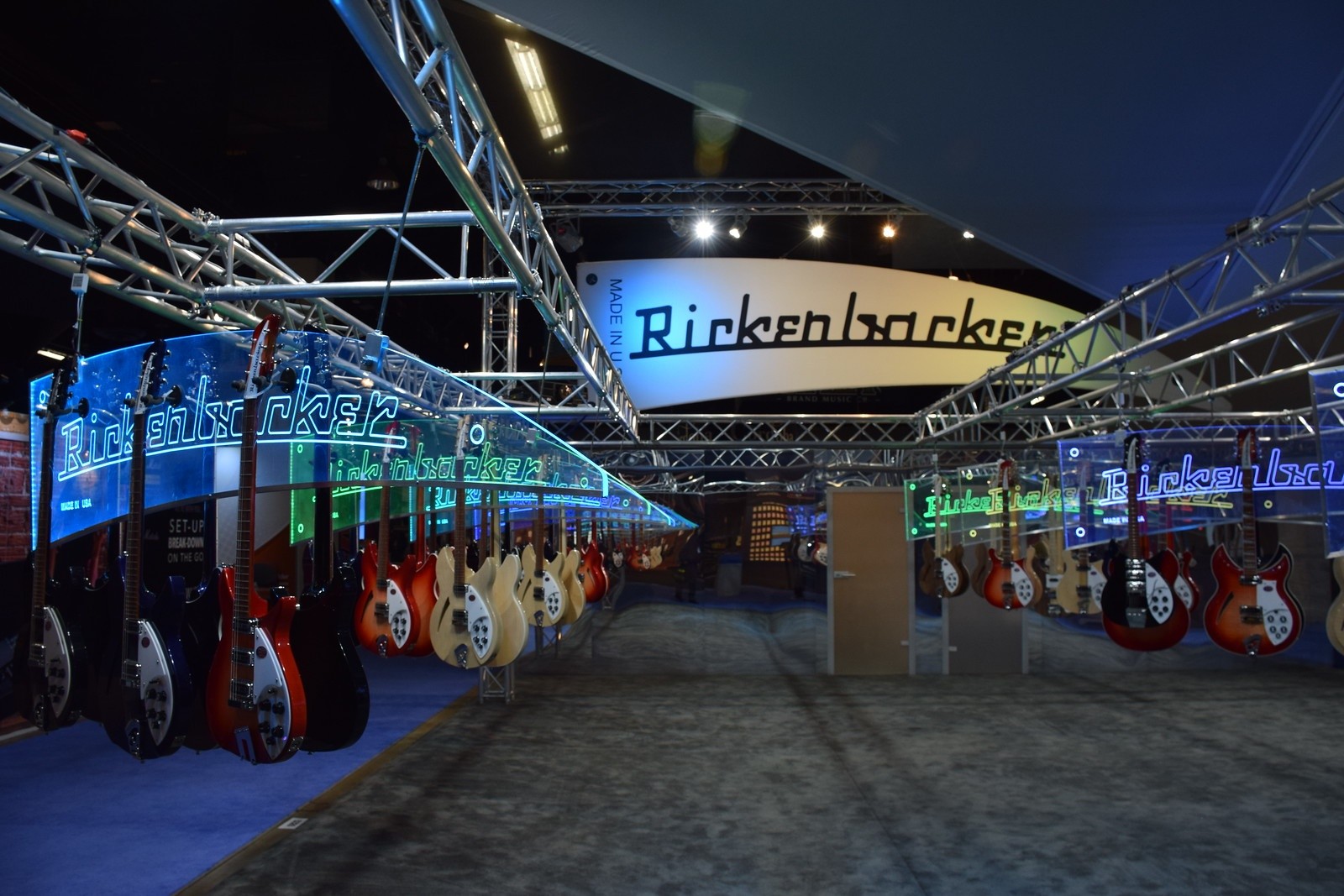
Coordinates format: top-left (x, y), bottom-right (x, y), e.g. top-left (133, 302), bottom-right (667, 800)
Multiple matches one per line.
top-left (1, 313), bottom-right (670, 764)
top-left (787, 426), bottom-right (1344, 656)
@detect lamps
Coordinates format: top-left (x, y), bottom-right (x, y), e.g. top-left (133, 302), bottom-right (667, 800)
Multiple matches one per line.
top-left (728, 209), bottom-right (751, 239)
top-left (366, 166), bottom-right (401, 191)
top-left (667, 211), bottom-right (689, 238)
top-left (882, 209), bottom-right (902, 236)
top-left (549, 215), bottom-right (583, 253)
